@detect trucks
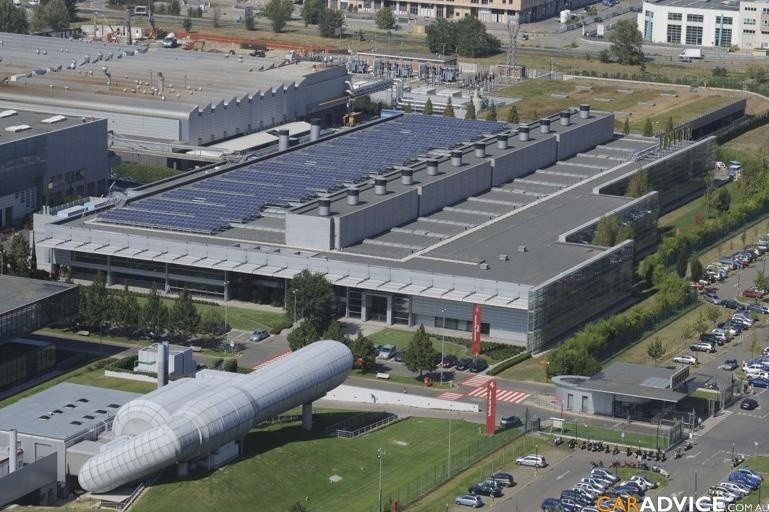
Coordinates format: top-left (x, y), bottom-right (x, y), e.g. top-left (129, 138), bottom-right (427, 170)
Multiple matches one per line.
top-left (678, 47), bottom-right (705, 59)
top-left (126, 4), bottom-right (149, 16)
top-left (12, 0), bottom-right (21, 8)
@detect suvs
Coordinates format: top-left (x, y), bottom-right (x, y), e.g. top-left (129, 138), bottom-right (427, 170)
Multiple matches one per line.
top-left (499, 413), bottom-right (521, 428)
top-left (514, 453), bottom-right (547, 469)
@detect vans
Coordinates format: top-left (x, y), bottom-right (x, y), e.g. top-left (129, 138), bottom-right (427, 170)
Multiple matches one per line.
top-left (188, 340), bottom-right (202, 352)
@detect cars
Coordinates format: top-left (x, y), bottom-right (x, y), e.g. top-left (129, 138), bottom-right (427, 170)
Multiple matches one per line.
top-left (249, 49), bottom-right (265, 57)
top-left (248, 327), bottom-right (270, 343)
top-left (537, 465), bottom-right (657, 512)
top-left (372, 342), bottom-right (490, 374)
top-left (722, 358), bottom-right (739, 371)
top-left (744, 346), bottom-right (769, 389)
top-left (582, 5), bottom-right (596, 13)
top-left (29, 0), bottom-right (40, 6)
top-left (688, 230), bottom-right (769, 354)
top-left (453, 470), bottom-right (516, 510)
top-left (602, 0), bottom-right (619, 6)
top-left (672, 353), bottom-right (699, 366)
top-left (739, 396), bottom-right (758, 410)
top-left (697, 466), bottom-right (764, 512)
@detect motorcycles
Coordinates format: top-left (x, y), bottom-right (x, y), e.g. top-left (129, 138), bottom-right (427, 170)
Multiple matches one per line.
top-left (553, 434), bottom-right (697, 465)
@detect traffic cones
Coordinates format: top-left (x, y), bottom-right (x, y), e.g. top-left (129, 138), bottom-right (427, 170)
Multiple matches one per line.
top-left (403, 386), bottom-right (408, 394)
top-left (374, 446), bottom-right (386, 512)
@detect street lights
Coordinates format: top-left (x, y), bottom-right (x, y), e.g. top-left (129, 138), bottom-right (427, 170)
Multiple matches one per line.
top-left (527, 10), bottom-right (532, 31)
top-left (223, 280), bottom-right (231, 362)
top-left (439, 305), bottom-right (448, 385)
top-left (445, 398), bottom-right (454, 482)
top-left (292, 288), bottom-right (299, 330)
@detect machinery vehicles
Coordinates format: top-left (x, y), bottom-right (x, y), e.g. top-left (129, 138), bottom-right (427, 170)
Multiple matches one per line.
top-left (162, 31), bottom-right (177, 49)
